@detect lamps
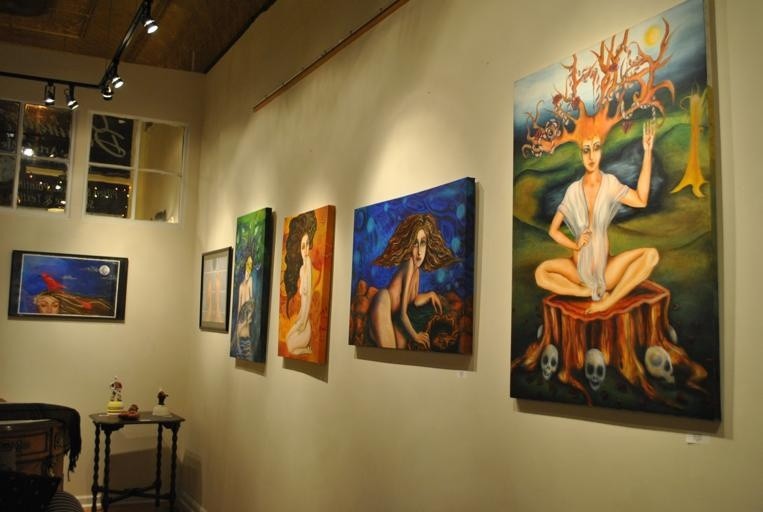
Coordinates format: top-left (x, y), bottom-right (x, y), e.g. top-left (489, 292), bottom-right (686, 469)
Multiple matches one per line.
top-left (100, 83), bottom-right (112, 100)
top-left (110, 74), bottom-right (124, 90)
top-left (43, 85), bottom-right (55, 106)
top-left (63, 87), bottom-right (79, 110)
top-left (140, 13), bottom-right (157, 34)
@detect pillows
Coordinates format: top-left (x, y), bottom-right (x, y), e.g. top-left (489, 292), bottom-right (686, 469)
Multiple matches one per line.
top-left (0, 469), bottom-right (62, 512)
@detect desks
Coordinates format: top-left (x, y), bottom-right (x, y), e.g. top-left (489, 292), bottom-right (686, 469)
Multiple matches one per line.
top-left (0, 403), bottom-right (78, 492)
top-left (88, 407), bottom-right (185, 511)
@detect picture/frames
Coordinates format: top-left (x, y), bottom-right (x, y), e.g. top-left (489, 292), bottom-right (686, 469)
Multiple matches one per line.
top-left (229, 208), bottom-right (274, 370)
top-left (510, 0), bottom-right (725, 419)
top-left (8, 249), bottom-right (129, 325)
top-left (196, 246), bottom-right (230, 333)
top-left (347, 177), bottom-right (475, 356)
top-left (276, 205), bottom-right (335, 366)
